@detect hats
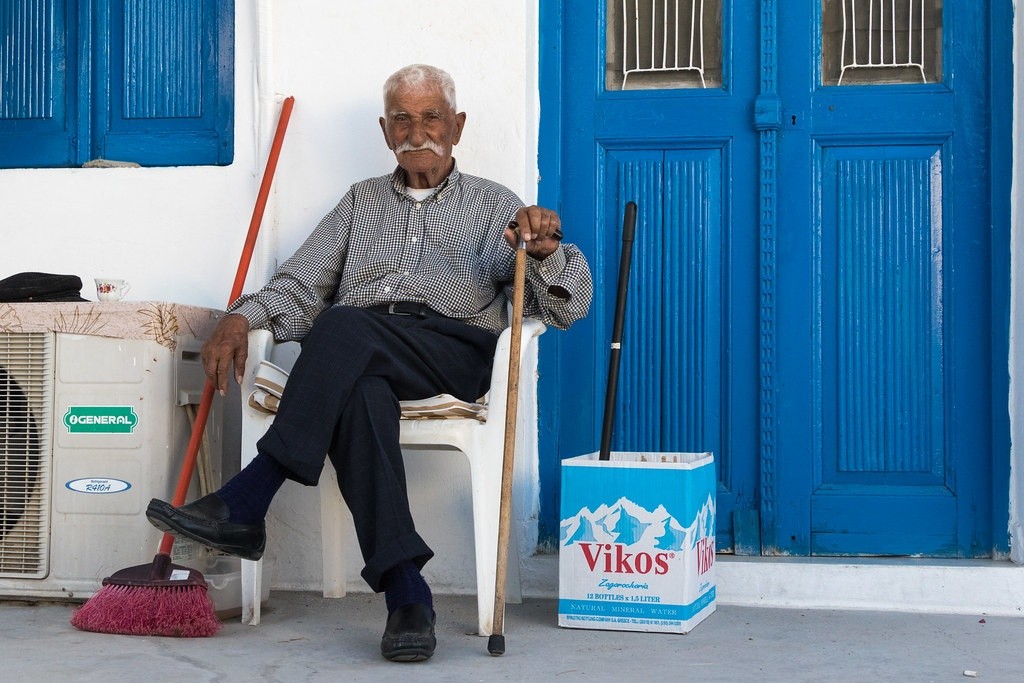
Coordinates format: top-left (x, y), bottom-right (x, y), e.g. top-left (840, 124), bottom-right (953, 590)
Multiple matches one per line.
top-left (0, 272), bottom-right (92, 303)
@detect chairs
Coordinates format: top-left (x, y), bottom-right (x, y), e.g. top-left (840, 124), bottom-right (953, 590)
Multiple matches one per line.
top-left (239, 317), bottom-right (546, 638)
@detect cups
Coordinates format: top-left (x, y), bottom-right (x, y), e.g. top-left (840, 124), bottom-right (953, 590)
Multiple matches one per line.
top-left (94, 278), bottom-right (131, 302)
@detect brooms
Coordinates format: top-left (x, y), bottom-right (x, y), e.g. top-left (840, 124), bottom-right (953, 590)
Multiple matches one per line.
top-left (67, 90), bottom-right (298, 639)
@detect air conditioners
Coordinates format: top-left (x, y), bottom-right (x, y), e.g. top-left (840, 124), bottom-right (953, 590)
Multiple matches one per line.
top-left (0, 303), bottom-right (271, 621)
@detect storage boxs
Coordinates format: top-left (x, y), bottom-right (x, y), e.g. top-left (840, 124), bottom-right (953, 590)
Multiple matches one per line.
top-left (557, 451), bottom-right (717, 635)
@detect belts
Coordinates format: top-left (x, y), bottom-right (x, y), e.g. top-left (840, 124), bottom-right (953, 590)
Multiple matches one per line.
top-left (368, 301), bottom-right (447, 318)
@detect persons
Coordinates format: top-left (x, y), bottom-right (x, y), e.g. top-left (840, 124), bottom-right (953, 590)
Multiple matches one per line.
top-left (145, 63), bottom-right (594, 662)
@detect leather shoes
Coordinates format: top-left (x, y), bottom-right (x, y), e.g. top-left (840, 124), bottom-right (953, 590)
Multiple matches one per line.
top-left (381, 602), bottom-right (436, 660)
top-left (145, 493), bottom-right (266, 561)
